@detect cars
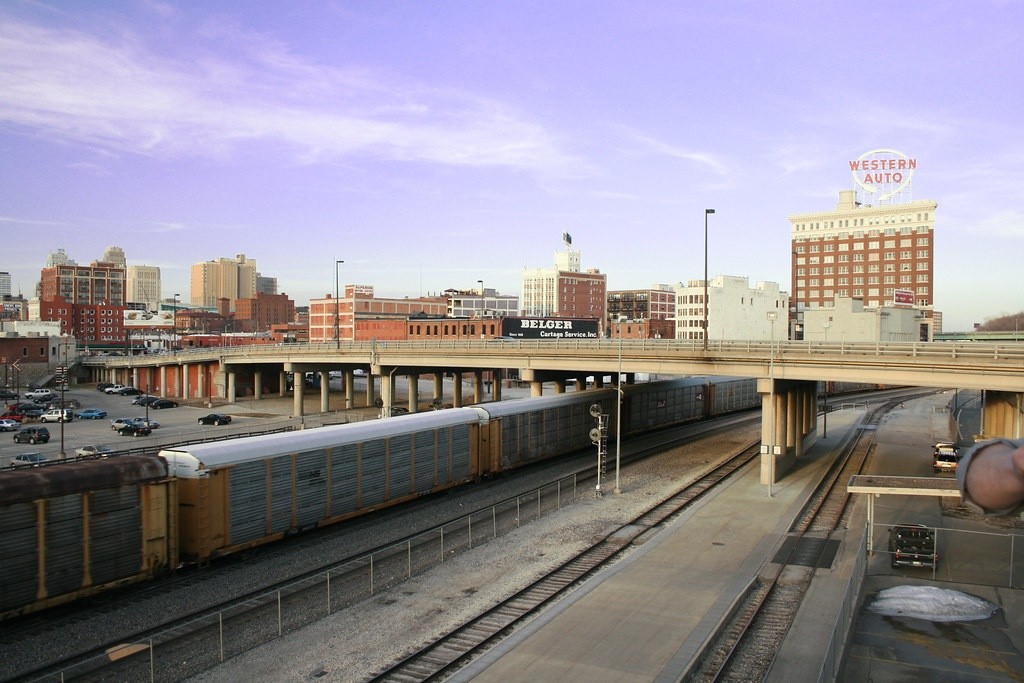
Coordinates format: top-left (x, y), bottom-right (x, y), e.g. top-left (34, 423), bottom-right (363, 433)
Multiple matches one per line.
top-left (9, 452), bottom-right (50, 471)
top-left (0, 401), bottom-right (47, 422)
top-left (284, 369), bottom-right (364, 392)
top-left (73, 408), bottom-right (107, 420)
top-left (150, 399), bottom-right (179, 410)
top-left (133, 416), bottom-right (161, 430)
top-left (96, 382), bottom-right (113, 392)
top-left (932, 440), bottom-right (959, 473)
top-left (118, 423), bottom-right (152, 438)
top-left (74, 444), bottom-right (117, 461)
top-left (196, 412), bottom-right (231, 426)
top-left (130, 394), bottom-right (144, 405)
top-left (0, 419), bottom-right (20, 432)
top-left (111, 418), bottom-right (132, 432)
top-left (118, 387), bottom-right (143, 396)
top-left (0, 390), bottom-right (22, 400)
top-left (141, 396), bottom-right (159, 406)
top-left (132, 344), bottom-right (149, 355)
top-left (55, 383), bottom-right (69, 392)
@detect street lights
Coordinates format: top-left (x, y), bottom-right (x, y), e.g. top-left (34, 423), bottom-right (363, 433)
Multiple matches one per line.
top-left (791, 250), bottom-right (799, 324)
top-left (172, 293), bottom-right (180, 357)
top-left (699, 207), bottom-right (716, 351)
top-left (477, 278), bottom-right (484, 316)
top-left (333, 260), bottom-right (345, 348)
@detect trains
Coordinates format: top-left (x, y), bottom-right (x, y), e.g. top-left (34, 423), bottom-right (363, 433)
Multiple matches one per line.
top-left (0, 374), bottom-right (915, 628)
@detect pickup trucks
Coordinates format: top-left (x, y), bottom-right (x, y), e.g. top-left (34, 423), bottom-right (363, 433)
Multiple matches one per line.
top-left (886, 522), bottom-right (939, 569)
top-left (105, 384), bottom-right (133, 394)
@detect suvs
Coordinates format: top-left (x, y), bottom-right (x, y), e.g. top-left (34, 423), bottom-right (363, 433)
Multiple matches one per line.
top-left (13, 425), bottom-right (51, 445)
top-left (25, 388), bottom-right (51, 399)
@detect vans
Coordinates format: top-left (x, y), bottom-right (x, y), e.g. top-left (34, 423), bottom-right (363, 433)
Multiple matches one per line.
top-left (40, 409), bottom-right (74, 422)
